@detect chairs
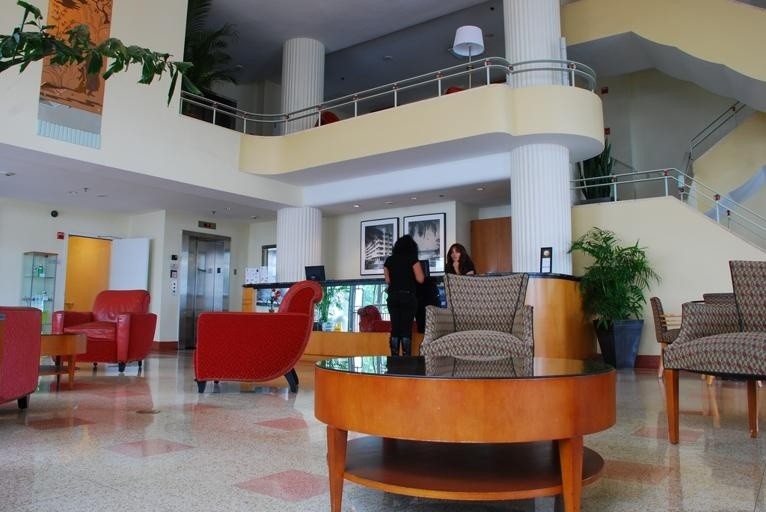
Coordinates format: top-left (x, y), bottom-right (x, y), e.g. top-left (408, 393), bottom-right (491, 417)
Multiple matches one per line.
top-left (0, 305), bottom-right (42, 409)
top-left (314, 111), bottom-right (339, 127)
top-left (649, 259), bottom-right (766, 444)
top-left (443, 86), bottom-right (463, 94)
top-left (417, 273), bottom-right (533, 360)
top-left (193, 281), bottom-right (323, 392)
top-left (52, 289), bottom-right (157, 372)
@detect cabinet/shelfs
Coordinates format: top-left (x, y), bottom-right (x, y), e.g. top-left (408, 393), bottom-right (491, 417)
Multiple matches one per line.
top-left (23, 251), bottom-right (58, 333)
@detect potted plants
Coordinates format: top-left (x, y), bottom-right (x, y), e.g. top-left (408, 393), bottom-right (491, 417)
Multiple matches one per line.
top-left (575, 136), bottom-right (613, 204)
top-left (565, 226), bottom-right (663, 368)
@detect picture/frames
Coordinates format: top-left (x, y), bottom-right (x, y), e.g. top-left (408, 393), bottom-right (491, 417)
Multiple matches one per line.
top-left (403, 212), bottom-right (447, 273)
top-left (359, 217), bottom-right (399, 276)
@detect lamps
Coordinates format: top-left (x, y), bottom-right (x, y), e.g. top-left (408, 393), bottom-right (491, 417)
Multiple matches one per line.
top-left (453, 25), bottom-right (484, 89)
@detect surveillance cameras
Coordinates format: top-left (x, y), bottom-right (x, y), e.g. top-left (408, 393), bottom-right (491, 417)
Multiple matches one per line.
top-left (51, 210), bottom-right (58, 217)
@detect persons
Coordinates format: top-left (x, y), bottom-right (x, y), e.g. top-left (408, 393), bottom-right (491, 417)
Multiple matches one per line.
top-left (380, 233), bottom-right (427, 357)
top-left (443, 242), bottom-right (478, 276)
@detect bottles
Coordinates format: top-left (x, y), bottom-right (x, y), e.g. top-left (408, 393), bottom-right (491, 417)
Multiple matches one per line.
top-left (33, 265), bottom-right (37, 276)
top-left (38, 300), bottom-right (48, 324)
top-left (38, 264), bottom-right (43, 275)
top-left (40, 291), bottom-right (48, 300)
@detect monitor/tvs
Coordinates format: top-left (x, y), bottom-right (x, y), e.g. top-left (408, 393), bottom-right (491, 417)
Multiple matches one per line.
top-left (305, 265), bottom-right (326, 281)
